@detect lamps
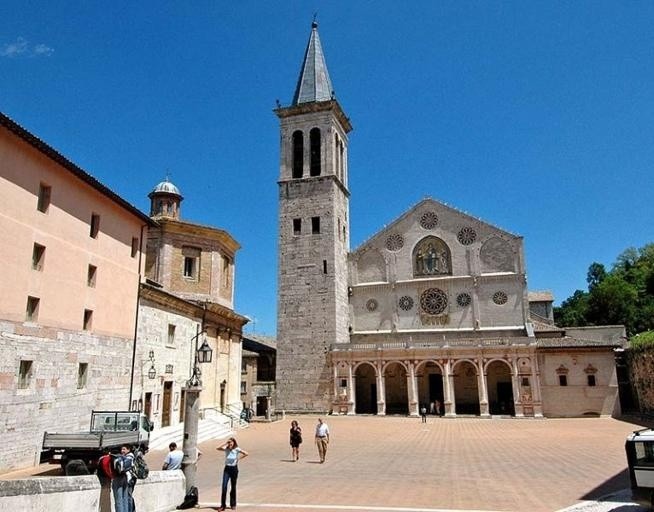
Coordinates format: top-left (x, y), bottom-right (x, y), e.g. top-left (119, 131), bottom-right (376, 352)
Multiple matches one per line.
top-left (190, 330), bottom-right (212, 364)
top-left (141, 358), bottom-right (156, 379)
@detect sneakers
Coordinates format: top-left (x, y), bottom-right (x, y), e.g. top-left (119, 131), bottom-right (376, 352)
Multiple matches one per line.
top-left (219, 507), bottom-right (225, 512)
top-left (232, 506), bottom-right (236, 510)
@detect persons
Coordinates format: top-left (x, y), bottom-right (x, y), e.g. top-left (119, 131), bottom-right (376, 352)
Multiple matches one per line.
top-left (160, 441), bottom-right (184, 469)
top-left (215, 437), bottom-right (248, 511)
top-left (420, 404), bottom-right (427, 422)
top-left (416, 243), bottom-right (447, 274)
top-left (434, 399), bottom-right (441, 414)
top-left (429, 400), bottom-right (434, 414)
top-left (196, 448), bottom-right (203, 461)
top-left (314, 416), bottom-right (329, 463)
top-left (94, 449), bottom-right (129, 511)
top-left (289, 419), bottom-right (302, 461)
top-left (119, 442), bottom-right (137, 511)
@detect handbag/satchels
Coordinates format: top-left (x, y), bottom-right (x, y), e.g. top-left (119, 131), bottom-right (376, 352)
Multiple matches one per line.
top-left (177, 485), bottom-right (198, 510)
top-left (111, 454), bottom-right (134, 474)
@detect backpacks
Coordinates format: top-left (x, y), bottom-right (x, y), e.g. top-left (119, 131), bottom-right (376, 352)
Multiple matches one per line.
top-left (127, 450), bottom-right (148, 479)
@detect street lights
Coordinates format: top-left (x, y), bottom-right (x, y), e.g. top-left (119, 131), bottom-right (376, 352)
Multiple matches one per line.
top-left (179, 330), bottom-right (214, 498)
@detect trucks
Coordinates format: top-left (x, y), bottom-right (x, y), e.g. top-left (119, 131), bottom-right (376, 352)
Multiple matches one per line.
top-left (34, 406), bottom-right (154, 475)
